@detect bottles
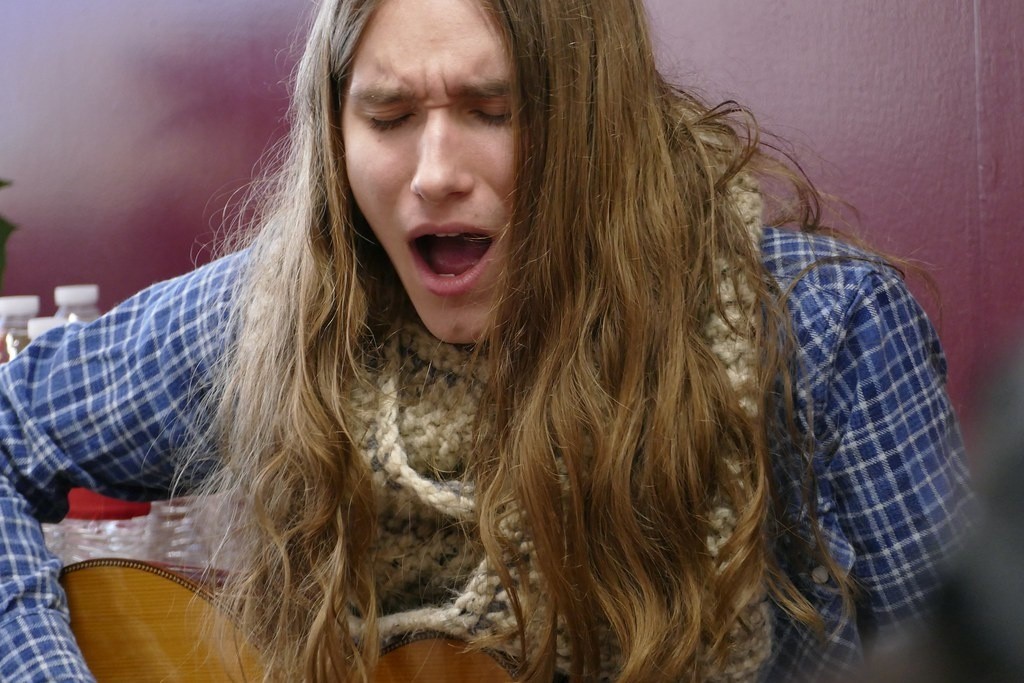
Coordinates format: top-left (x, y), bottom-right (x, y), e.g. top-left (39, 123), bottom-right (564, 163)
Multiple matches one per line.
top-left (0, 281), bottom-right (210, 568)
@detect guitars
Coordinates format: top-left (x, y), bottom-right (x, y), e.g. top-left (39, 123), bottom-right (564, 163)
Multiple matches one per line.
top-left (50, 558), bottom-right (520, 683)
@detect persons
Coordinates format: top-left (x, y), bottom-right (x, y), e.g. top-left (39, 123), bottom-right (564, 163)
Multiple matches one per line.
top-left (0, 0), bottom-right (984, 683)
